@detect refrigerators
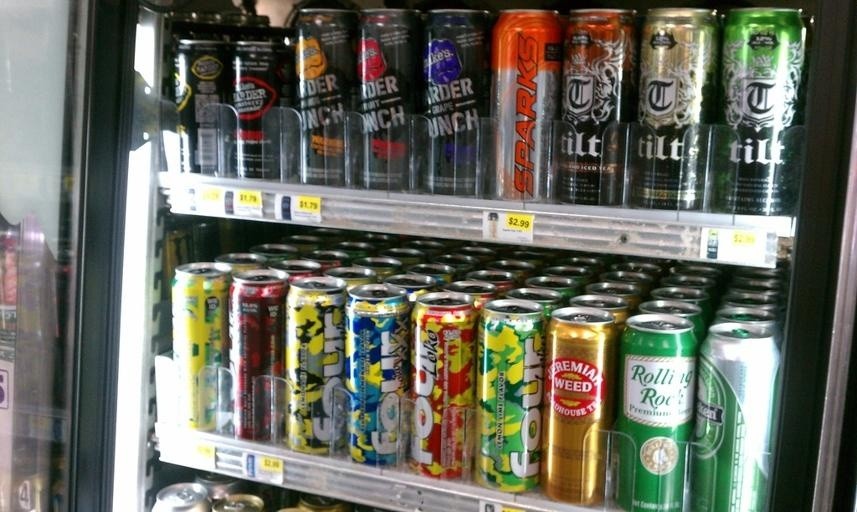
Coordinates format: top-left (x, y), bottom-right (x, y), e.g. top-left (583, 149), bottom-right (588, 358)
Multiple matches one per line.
top-left (49, 0), bottom-right (855, 512)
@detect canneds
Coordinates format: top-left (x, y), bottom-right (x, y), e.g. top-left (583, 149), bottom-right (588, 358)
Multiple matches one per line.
top-left (172, 40), bottom-right (226, 174)
top-left (620, 313), bottom-right (698, 512)
top-left (630, 6), bottom-right (719, 212)
top-left (230, 41), bottom-right (291, 179)
top-left (286, 275), bottom-right (348, 456)
top-left (293, 8), bottom-right (355, 186)
top-left (171, 261), bottom-right (233, 434)
top-left (210, 494), bottom-right (265, 511)
top-left (356, 7), bottom-right (425, 191)
top-left (345, 284), bottom-right (411, 468)
top-left (424, 9), bottom-right (490, 195)
top-left (214, 226), bottom-right (786, 324)
top-left (195, 473), bottom-right (239, 499)
top-left (693, 323), bottom-right (785, 510)
top-left (150, 481), bottom-right (211, 512)
top-left (298, 495), bottom-right (344, 512)
top-left (475, 299), bottom-right (546, 494)
top-left (411, 291), bottom-right (477, 479)
top-left (229, 269), bottom-right (289, 444)
top-left (722, 7), bottom-right (809, 216)
top-left (494, 8), bottom-right (560, 202)
top-left (544, 307), bottom-right (620, 507)
top-left (554, 9), bottom-right (636, 208)
